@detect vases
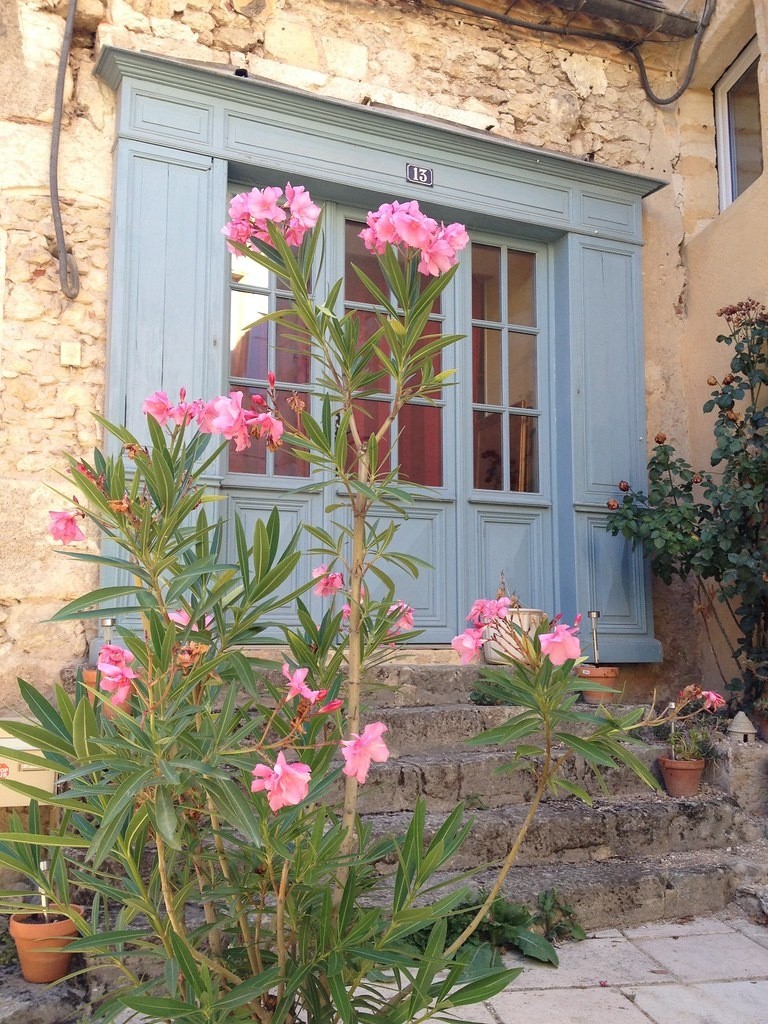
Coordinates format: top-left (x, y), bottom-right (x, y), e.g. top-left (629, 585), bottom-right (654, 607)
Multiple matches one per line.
top-left (576, 667), bottom-right (618, 705)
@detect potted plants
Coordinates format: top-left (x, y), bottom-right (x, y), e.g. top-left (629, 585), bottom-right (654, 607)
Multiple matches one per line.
top-left (0, 799), bottom-right (85, 983)
top-left (477, 569), bottom-right (545, 664)
top-left (655, 718), bottom-right (729, 797)
